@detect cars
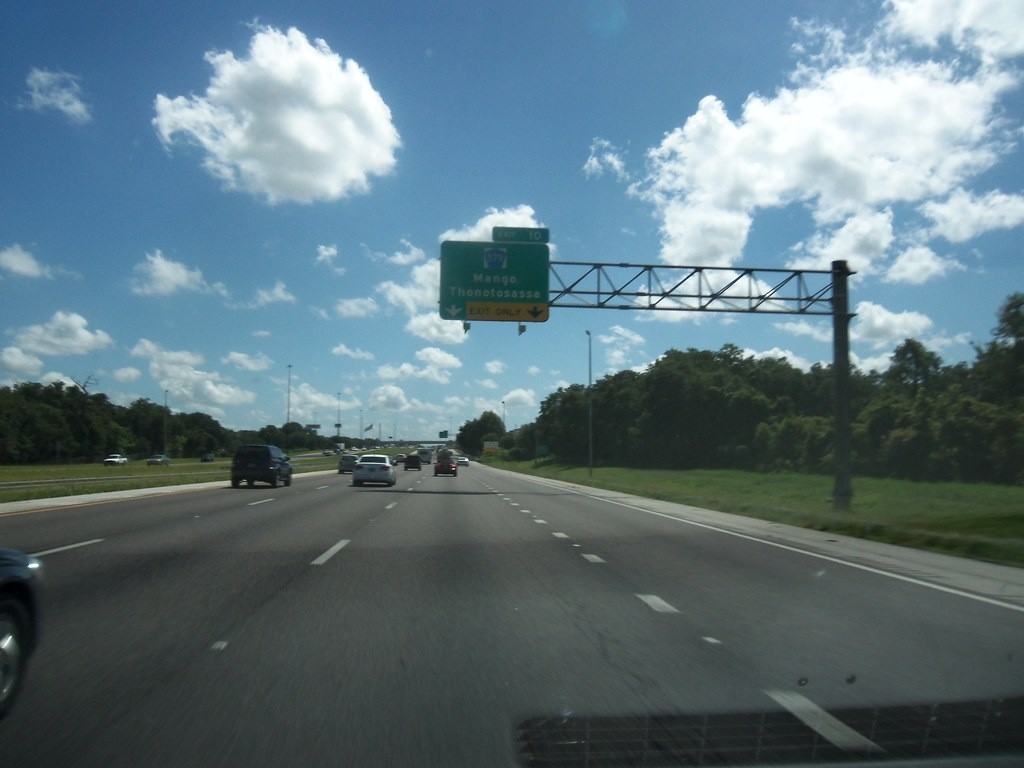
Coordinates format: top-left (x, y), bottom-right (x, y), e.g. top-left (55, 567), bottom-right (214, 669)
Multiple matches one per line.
top-left (456, 457), bottom-right (469, 467)
top-left (201, 453), bottom-right (215, 462)
top-left (352, 454), bottom-right (398, 487)
top-left (146, 454), bottom-right (170, 466)
top-left (338, 454), bottom-right (359, 474)
top-left (322, 440), bottom-right (452, 466)
top-left (0, 546), bottom-right (49, 715)
top-left (403, 454), bottom-right (423, 471)
top-left (433, 460), bottom-right (457, 476)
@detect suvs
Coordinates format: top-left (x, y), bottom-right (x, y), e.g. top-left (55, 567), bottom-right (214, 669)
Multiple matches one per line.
top-left (230, 444), bottom-right (293, 488)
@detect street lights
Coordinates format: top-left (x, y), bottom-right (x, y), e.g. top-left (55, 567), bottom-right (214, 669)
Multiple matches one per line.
top-left (164, 389), bottom-right (169, 457)
top-left (584, 329), bottom-right (594, 477)
top-left (501, 400), bottom-right (505, 424)
top-left (337, 392), bottom-right (342, 442)
top-left (286, 363), bottom-right (293, 457)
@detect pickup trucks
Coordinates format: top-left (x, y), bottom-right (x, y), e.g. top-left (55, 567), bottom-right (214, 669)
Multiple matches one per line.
top-left (103, 453), bottom-right (128, 466)
